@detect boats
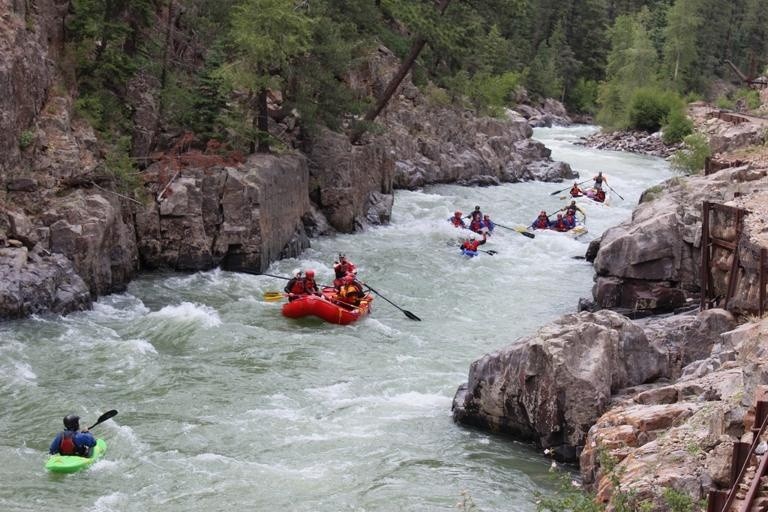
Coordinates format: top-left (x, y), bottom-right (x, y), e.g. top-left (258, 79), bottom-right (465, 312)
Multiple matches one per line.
top-left (281, 288), bottom-right (371, 325)
top-left (462, 185), bottom-right (606, 258)
top-left (44, 437), bottom-right (108, 475)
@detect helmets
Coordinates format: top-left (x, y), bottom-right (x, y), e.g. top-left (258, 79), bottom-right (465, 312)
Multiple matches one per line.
top-left (537, 200), bottom-right (577, 220)
top-left (453, 205), bottom-right (489, 240)
top-left (573, 169), bottom-right (606, 193)
top-left (63, 414), bottom-right (82, 431)
top-left (292, 252), bottom-right (353, 285)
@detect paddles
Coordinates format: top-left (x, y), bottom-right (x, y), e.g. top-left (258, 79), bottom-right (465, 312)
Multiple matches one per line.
top-left (88, 410), bottom-right (117, 430)
top-left (477, 249), bottom-right (496, 256)
top-left (363, 283), bottom-right (420, 321)
top-left (493, 222), bottom-right (534, 239)
top-left (560, 191), bottom-right (582, 200)
top-left (607, 185), bottom-right (624, 201)
top-left (263, 291), bottom-right (312, 302)
top-left (550, 178), bottom-right (593, 196)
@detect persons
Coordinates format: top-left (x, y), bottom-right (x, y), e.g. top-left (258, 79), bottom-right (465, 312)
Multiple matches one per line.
top-left (593, 171), bottom-right (608, 188)
top-left (482, 214), bottom-right (494, 232)
top-left (447, 210), bottom-right (467, 229)
top-left (554, 214), bottom-right (569, 232)
top-left (561, 200), bottom-right (584, 219)
top-left (49, 413), bottom-right (97, 458)
top-left (532, 210), bottom-right (550, 229)
top-left (469, 214), bottom-right (485, 231)
top-left (563, 209), bottom-right (576, 228)
top-left (460, 230), bottom-right (486, 252)
top-left (282, 250), bottom-right (365, 310)
top-left (570, 183), bottom-right (582, 196)
top-left (468, 206), bottom-right (483, 219)
